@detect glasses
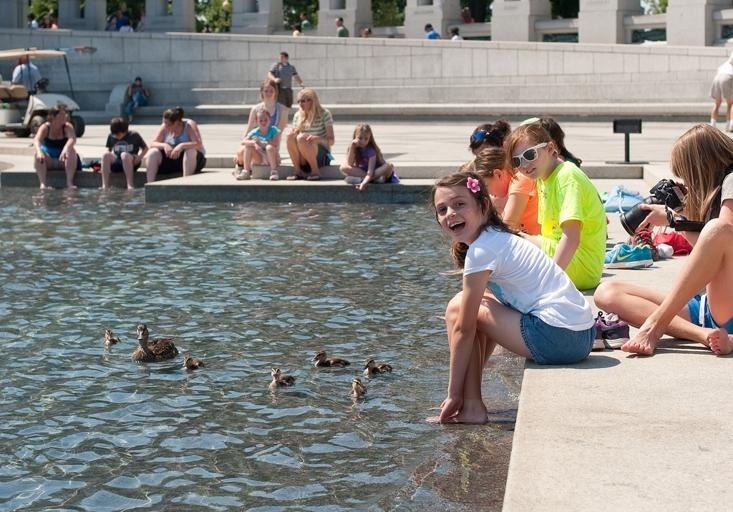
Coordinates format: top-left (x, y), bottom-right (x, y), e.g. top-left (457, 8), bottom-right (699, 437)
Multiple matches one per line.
top-left (511, 142), bottom-right (549, 169)
top-left (297, 99), bottom-right (305, 103)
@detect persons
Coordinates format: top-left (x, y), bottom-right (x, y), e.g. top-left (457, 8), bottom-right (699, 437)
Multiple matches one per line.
top-left (422, 170), bottom-right (598, 424)
top-left (100, 117), bottom-right (150, 192)
top-left (334, 16), bottom-right (349, 37)
top-left (234, 78), bottom-right (289, 178)
top-left (594, 120), bottom-right (733, 360)
top-left (11, 52), bottom-right (48, 96)
top-left (503, 125), bottom-right (608, 292)
top-left (267, 52), bottom-right (306, 129)
top-left (233, 108), bottom-right (281, 182)
top-left (459, 117), bottom-right (582, 237)
top-left (298, 10), bottom-right (312, 31)
top-left (339, 122), bottom-right (395, 192)
top-left (125, 76), bottom-right (150, 124)
top-left (709, 52), bottom-right (733, 133)
top-left (14, 6), bottom-right (64, 32)
top-left (285, 87), bottom-right (335, 180)
top-left (33, 103), bottom-right (80, 189)
top-left (447, 25), bottom-right (462, 40)
top-left (461, 6), bottom-right (474, 24)
top-left (144, 106), bottom-right (207, 201)
top-left (424, 22), bottom-right (440, 39)
top-left (104, 4), bottom-right (147, 36)
top-left (292, 30), bottom-right (300, 36)
top-left (360, 27), bottom-right (370, 38)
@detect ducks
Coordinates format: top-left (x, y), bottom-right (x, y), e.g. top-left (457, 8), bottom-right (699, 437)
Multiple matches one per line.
top-left (104, 329), bottom-right (120, 343)
top-left (310, 350), bottom-right (351, 369)
top-left (363, 357), bottom-right (393, 375)
top-left (348, 377), bottom-right (368, 398)
top-left (182, 353), bottom-right (205, 370)
top-left (269, 367), bottom-right (296, 387)
top-left (131, 324), bottom-right (179, 362)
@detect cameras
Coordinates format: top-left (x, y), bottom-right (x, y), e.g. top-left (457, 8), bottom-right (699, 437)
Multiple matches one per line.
top-left (619, 178), bottom-right (688, 236)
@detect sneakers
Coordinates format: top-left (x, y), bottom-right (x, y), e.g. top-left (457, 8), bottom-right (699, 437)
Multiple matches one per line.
top-left (603, 243), bottom-right (642, 270)
top-left (641, 244), bottom-right (653, 267)
top-left (628, 228), bottom-right (659, 263)
top-left (591, 310), bottom-right (630, 350)
top-left (237, 169), bottom-right (252, 180)
top-left (269, 169), bottom-right (279, 179)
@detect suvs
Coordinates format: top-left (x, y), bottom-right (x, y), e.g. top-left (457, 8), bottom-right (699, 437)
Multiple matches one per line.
top-left (0, 44), bottom-right (85, 141)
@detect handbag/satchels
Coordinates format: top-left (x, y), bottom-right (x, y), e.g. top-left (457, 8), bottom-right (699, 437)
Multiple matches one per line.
top-left (604, 185), bottom-right (645, 212)
top-left (652, 230), bottom-right (692, 256)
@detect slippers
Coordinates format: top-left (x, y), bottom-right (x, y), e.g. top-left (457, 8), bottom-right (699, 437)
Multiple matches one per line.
top-left (306, 173), bottom-right (321, 181)
top-left (286, 174), bottom-right (307, 180)
